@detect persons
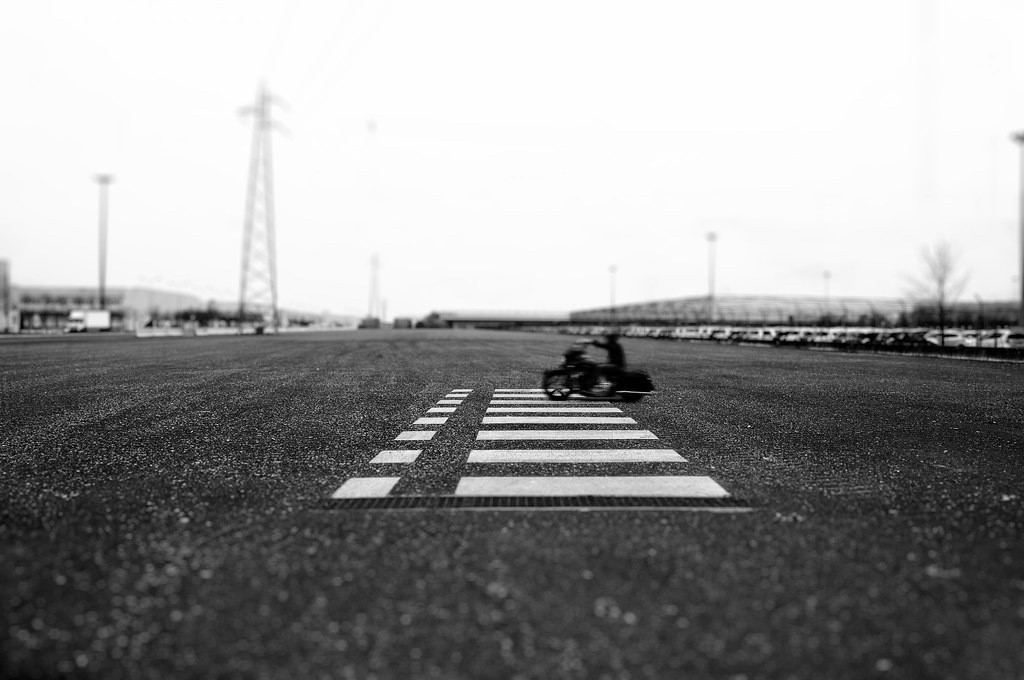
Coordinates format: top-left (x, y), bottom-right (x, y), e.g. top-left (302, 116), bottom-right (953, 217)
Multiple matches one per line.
top-left (573, 330), bottom-right (626, 390)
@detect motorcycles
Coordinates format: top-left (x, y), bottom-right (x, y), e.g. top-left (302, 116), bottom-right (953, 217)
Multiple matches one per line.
top-left (542, 338), bottom-right (661, 404)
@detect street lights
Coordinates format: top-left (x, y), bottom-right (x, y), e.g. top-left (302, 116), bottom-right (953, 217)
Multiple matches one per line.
top-left (707, 231), bottom-right (718, 324)
top-left (607, 267), bottom-right (620, 333)
top-left (95, 176), bottom-right (112, 310)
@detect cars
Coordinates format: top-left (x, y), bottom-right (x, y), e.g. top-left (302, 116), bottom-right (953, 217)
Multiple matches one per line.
top-left (522, 324), bottom-right (1024, 350)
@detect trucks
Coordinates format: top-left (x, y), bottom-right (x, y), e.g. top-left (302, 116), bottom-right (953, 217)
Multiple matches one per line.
top-left (66, 310), bottom-right (111, 332)
